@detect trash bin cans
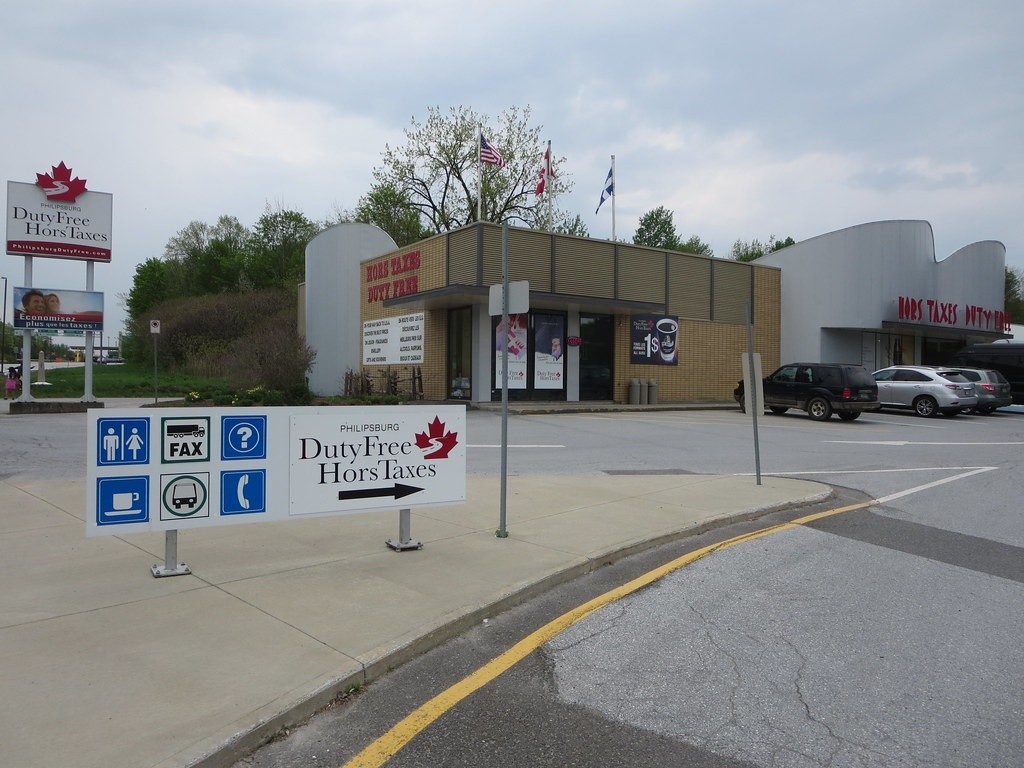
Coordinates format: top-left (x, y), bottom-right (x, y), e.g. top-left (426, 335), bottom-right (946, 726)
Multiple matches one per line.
top-left (640, 379), bottom-right (648, 404)
top-left (628, 378), bottom-right (640, 404)
top-left (648, 378), bottom-right (658, 403)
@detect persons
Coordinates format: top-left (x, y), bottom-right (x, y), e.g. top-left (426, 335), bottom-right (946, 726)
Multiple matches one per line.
top-left (14, 362), bottom-right (35, 390)
top-left (2, 367), bottom-right (17, 400)
top-left (21, 290), bottom-right (45, 315)
top-left (44, 293), bottom-right (60, 313)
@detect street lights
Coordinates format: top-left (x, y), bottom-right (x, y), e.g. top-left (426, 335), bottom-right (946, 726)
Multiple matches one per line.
top-left (1, 277), bottom-right (7, 374)
top-left (107, 336), bottom-right (110, 356)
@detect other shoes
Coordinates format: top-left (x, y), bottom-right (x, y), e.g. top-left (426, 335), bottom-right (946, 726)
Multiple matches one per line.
top-left (4, 398), bottom-right (7, 400)
top-left (12, 398), bottom-right (14, 400)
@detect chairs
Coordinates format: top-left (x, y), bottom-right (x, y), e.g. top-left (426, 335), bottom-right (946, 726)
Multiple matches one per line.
top-left (803, 372), bottom-right (809, 383)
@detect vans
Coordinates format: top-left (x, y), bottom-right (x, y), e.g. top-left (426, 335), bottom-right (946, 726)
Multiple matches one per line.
top-left (935, 342), bottom-right (1024, 404)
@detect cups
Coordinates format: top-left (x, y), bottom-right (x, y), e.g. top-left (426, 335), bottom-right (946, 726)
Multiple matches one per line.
top-left (656, 319), bottom-right (678, 361)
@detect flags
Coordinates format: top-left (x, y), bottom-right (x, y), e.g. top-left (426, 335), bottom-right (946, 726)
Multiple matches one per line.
top-left (480, 134), bottom-right (505, 167)
top-left (536, 147), bottom-right (556, 196)
top-left (595, 167), bottom-right (613, 215)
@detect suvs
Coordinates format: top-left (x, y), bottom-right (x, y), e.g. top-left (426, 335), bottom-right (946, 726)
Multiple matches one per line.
top-left (861, 364), bottom-right (978, 418)
top-left (940, 367), bottom-right (1013, 414)
top-left (733, 362), bottom-right (880, 421)
